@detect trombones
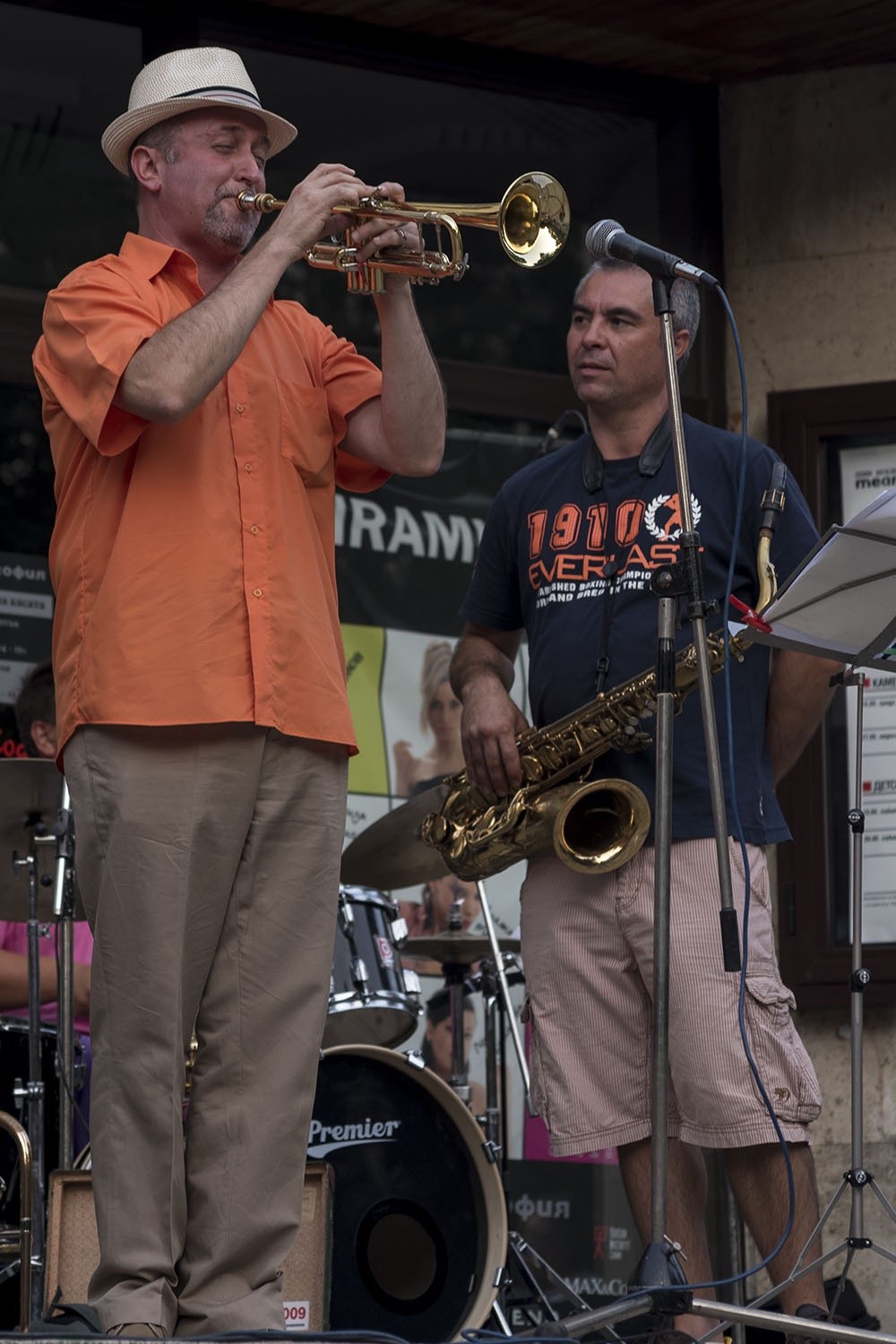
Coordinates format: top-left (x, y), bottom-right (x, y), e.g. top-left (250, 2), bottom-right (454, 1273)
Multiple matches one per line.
top-left (1, 1109), bottom-right (34, 1335)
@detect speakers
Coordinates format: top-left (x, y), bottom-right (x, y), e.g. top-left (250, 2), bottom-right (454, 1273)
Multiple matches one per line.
top-left (48, 1159), bottom-right (335, 1344)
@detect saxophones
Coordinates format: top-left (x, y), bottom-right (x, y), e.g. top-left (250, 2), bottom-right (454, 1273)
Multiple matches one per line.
top-left (423, 456), bottom-right (794, 884)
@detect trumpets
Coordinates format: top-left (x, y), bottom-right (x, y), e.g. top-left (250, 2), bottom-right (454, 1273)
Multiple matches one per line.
top-left (237, 166), bottom-right (574, 299)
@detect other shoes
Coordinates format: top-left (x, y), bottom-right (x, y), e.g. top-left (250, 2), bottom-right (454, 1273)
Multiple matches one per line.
top-left (784, 1303), bottom-right (839, 1344)
top-left (106, 1321), bottom-right (168, 1338)
top-left (661, 1330), bottom-right (734, 1344)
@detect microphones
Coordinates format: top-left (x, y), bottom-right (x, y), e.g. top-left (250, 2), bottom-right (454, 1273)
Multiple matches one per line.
top-left (586, 219), bottom-right (721, 290)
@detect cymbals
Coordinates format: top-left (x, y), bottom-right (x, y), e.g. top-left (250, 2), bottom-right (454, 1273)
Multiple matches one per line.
top-left (2, 753), bottom-right (88, 926)
top-left (402, 927), bottom-right (522, 963)
top-left (338, 781), bottom-right (453, 890)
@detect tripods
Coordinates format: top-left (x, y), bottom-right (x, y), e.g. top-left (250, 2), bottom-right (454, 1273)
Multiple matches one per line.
top-left (479, 280), bottom-right (896, 1344)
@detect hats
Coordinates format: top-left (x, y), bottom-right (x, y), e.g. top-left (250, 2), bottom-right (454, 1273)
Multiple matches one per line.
top-left (100, 47), bottom-right (298, 176)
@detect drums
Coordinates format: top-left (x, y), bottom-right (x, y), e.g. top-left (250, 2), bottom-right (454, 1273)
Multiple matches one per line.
top-left (318, 884), bottom-right (420, 1049)
top-left (74, 1039), bottom-right (510, 1342)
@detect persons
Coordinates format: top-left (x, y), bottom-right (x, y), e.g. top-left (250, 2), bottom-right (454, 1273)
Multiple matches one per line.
top-left (29, 45), bottom-right (447, 1343)
top-left (394, 640), bottom-right (467, 797)
top-left (450, 258), bottom-right (877, 1344)
top-left (420, 991), bottom-right (486, 1133)
top-left (397, 871), bottom-right (480, 975)
top-left (0, 663), bottom-right (191, 1173)
